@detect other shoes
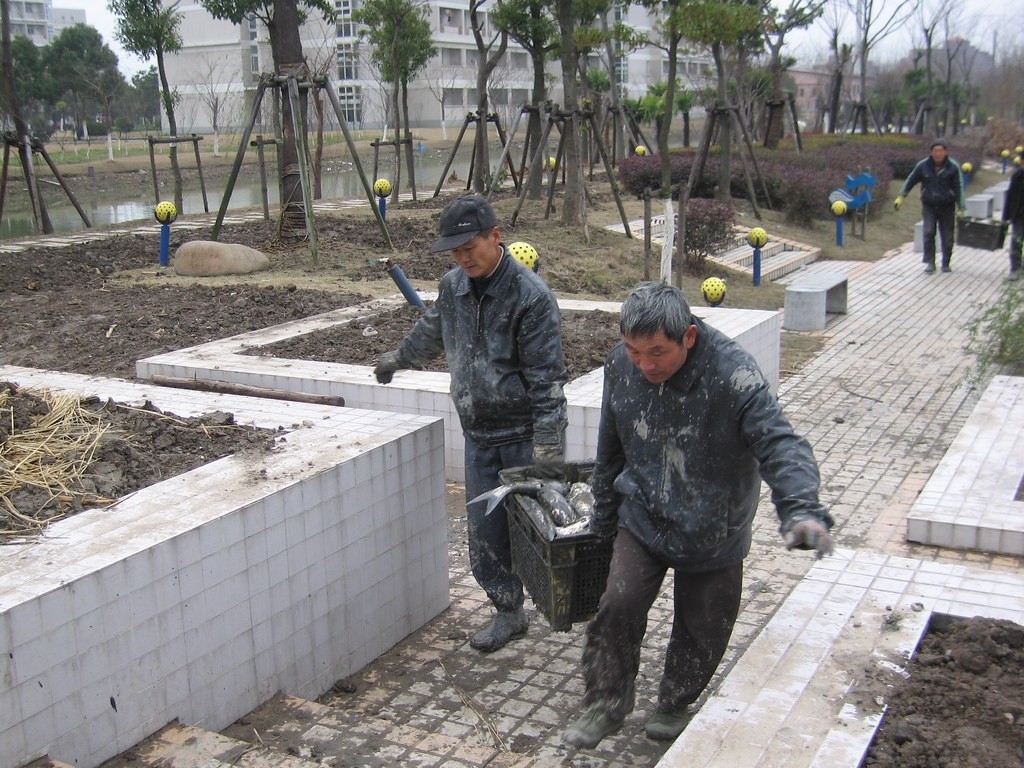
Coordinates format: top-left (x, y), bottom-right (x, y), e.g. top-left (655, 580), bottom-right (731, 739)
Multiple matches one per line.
top-left (942, 266), bottom-right (952, 273)
top-left (924, 264), bottom-right (936, 274)
top-left (1008, 268), bottom-right (1020, 280)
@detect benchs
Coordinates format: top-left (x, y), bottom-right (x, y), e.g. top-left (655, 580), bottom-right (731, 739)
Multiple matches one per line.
top-left (785, 273), bottom-right (849, 328)
top-left (913, 221), bottom-right (941, 253)
top-left (966, 194), bottom-right (995, 218)
top-left (984, 181), bottom-right (1011, 210)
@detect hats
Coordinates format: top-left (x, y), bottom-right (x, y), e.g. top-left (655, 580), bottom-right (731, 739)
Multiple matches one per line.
top-left (431, 195), bottom-right (499, 252)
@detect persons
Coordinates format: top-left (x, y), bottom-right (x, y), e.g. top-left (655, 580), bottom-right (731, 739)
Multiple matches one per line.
top-left (373, 195), bottom-right (569, 653)
top-left (562, 279), bottom-right (836, 750)
top-left (893, 139), bottom-right (967, 272)
top-left (1002, 150), bottom-right (1024, 281)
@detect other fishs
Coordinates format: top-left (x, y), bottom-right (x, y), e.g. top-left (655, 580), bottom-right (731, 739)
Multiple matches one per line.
top-left (464, 475), bottom-right (595, 542)
top-left (964, 216), bottom-right (1002, 229)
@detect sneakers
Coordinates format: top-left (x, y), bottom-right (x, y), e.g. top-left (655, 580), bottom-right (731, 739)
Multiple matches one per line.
top-left (470, 604), bottom-right (528, 654)
top-left (644, 708), bottom-right (689, 738)
top-left (561, 706), bottom-right (625, 749)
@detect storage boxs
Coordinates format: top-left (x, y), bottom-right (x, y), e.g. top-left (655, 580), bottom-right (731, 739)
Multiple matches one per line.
top-left (498, 458), bottom-right (619, 630)
top-left (956, 215), bottom-right (1009, 252)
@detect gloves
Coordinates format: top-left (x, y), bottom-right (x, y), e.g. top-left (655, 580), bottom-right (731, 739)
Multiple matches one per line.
top-left (956, 211), bottom-right (963, 216)
top-left (374, 351), bottom-right (404, 384)
top-left (531, 444), bottom-right (566, 464)
top-left (894, 196), bottom-right (904, 211)
